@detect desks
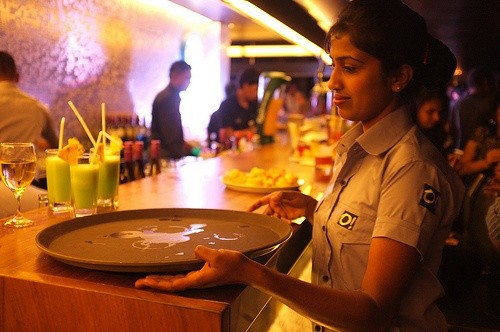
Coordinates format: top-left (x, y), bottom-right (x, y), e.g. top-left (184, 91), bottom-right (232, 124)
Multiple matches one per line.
top-left (0, 129), bottom-right (338, 332)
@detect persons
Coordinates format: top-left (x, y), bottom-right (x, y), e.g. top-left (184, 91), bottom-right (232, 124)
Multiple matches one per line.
top-left (398, 34), bottom-right (500, 332)
top-left (134, 0), bottom-right (458, 332)
top-left (149, 59), bottom-right (195, 159)
top-left (0, 51), bottom-right (60, 168)
top-left (207, 67), bottom-right (308, 151)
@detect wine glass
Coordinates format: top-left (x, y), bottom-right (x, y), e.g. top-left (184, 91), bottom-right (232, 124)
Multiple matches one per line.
top-left (0, 142), bottom-right (37, 228)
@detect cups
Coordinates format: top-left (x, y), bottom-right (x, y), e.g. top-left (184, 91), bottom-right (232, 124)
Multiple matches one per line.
top-left (90, 142), bottom-right (120, 208)
top-left (69, 152), bottom-right (100, 218)
top-left (45, 148), bottom-right (74, 213)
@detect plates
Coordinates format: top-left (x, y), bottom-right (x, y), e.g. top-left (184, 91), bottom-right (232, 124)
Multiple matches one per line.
top-left (220, 174), bottom-right (304, 192)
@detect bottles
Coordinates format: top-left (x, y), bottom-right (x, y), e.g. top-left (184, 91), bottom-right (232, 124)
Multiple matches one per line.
top-left (119, 139), bottom-right (161, 185)
